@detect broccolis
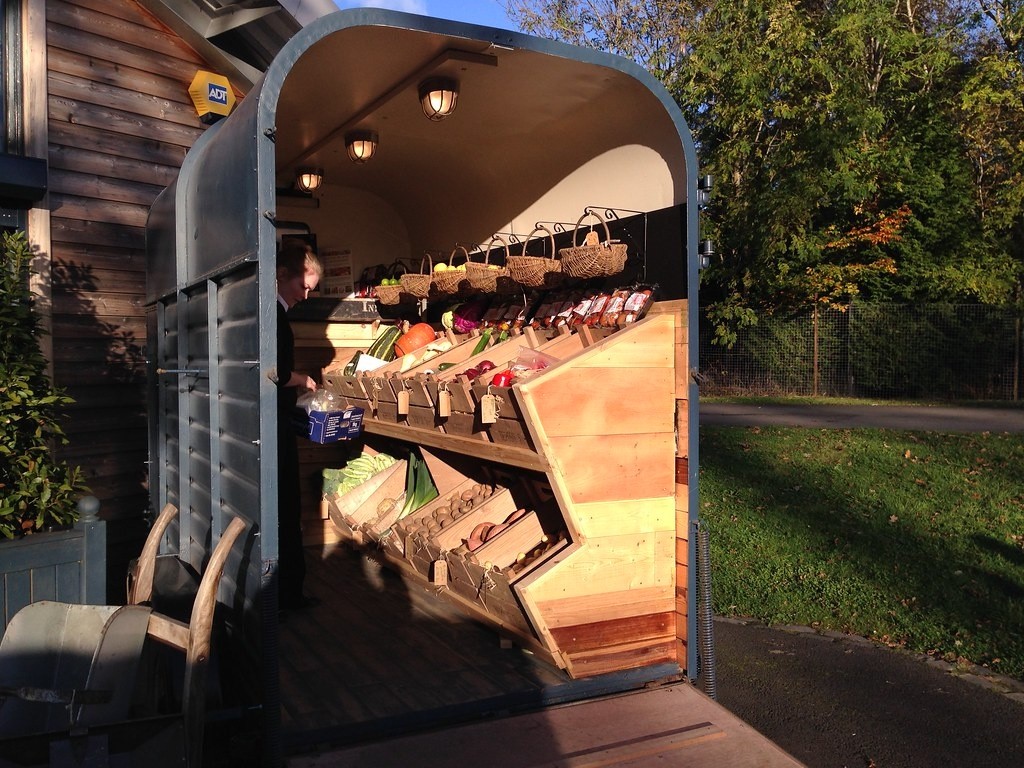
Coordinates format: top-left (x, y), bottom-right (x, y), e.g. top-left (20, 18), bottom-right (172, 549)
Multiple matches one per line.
top-left (320, 467), bottom-right (346, 496)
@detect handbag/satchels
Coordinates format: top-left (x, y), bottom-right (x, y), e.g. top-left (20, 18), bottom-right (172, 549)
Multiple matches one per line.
top-left (354, 262), bottom-right (417, 298)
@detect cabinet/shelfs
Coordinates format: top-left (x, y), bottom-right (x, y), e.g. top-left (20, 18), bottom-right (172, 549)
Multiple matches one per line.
top-left (315, 313), bottom-right (677, 680)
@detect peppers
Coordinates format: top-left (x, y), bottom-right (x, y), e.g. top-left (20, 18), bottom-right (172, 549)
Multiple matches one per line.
top-left (491, 369), bottom-right (517, 388)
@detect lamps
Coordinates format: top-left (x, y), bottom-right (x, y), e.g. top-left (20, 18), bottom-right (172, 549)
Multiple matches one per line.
top-left (416, 76), bottom-right (460, 122)
top-left (294, 166), bottom-right (324, 194)
top-left (343, 129), bottom-right (379, 165)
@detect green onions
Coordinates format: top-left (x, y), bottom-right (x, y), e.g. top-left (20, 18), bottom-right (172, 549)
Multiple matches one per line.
top-left (383, 451), bottom-right (439, 537)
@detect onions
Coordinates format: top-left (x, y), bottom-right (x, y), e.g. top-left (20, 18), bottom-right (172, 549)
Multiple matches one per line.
top-left (452, 360), bottom-right (496, 383)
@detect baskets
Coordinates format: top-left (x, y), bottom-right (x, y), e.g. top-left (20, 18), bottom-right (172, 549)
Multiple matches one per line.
top-left (375, 236), bottom-right (509, 305)
top-left (559, 213), bottom-right (628, 279)
top-left (507, 228), bottom-right (564, 290)
top-left (318, 248), bottom-right (354, 298)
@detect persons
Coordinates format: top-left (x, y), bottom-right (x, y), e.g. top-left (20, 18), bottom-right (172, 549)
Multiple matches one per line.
top-left (275, 239), bottom-right (324, 611)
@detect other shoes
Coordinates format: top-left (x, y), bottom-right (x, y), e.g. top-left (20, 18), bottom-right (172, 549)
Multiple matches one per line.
top-left (282, 595), bottom-right (321, 610)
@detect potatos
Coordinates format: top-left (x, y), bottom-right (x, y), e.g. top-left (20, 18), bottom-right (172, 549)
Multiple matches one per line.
top-left (511, 528), bottom-right (569, 574)
top-left (403, 479), bottom-right (506, 536)
top-left (471, 507), bottom-right (532, 542)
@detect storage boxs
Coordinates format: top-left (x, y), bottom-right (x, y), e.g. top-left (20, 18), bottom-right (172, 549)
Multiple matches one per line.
top-left (463, 510), bottom-right (571, 638)
top-left (296, 406), bottom-right (365, 445)
top-left (343, 458), bottom-right (408, 544)
top-left (389, 328), bottom-right (521, 434)
top-left (361, 330), bottom-right (481, 427)
top-left (471, 321), bottom-right (631, 450)
top-left (427, 485), bottom-right (552, 614)
top-left (426, 325), bottom-right (571, 441)
top-left (337, 331), bottom-right (444, 420)
top-left (367, 497), bottom-right (411, 565)
top-left (322, 348), bottom-right (368, 409)
top-left (321, 456), bottom-right (404, 535)
top-left (390, 469), bottom-right (514, 591)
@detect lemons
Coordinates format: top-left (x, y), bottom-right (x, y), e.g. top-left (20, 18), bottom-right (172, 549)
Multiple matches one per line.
top-left (380, 278), bottom-right (401, 285)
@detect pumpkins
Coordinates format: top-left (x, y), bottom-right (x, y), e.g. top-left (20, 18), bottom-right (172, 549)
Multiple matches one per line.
top-left (344, 322), bottom-right (437, 376)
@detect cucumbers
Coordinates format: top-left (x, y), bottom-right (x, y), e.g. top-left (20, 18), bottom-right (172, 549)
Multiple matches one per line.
top-left (438, 327), bottom-right (508, 370)
